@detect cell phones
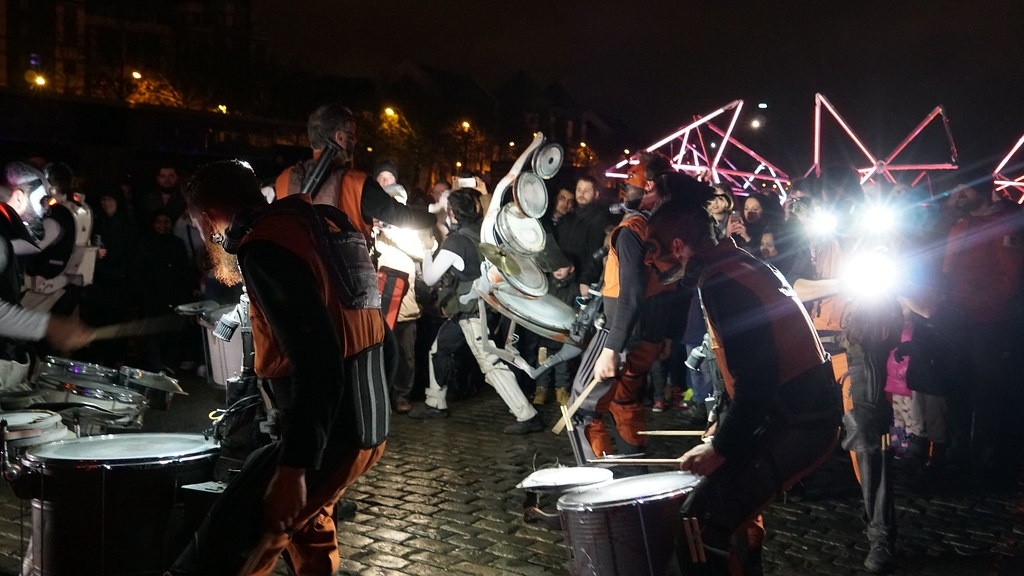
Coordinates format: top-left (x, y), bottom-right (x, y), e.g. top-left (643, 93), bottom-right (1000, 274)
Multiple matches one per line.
top-left (458, 178), bottom-right (476, 188)
top-left (731, 210), bottom-right (741, 224)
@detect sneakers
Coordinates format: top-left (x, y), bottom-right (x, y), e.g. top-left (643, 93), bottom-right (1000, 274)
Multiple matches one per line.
top-left (534, 386), bottom-right (548, 405)
top-left (556, 387), bottom-right (571, 404)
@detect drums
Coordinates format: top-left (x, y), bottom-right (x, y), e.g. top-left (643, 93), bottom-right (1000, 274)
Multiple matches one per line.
top-left (530, 142), bottom-right (564, 179)
top-left (553, 469), bottom-right (704, 576)
top-left (30, 353), bottom-right (119, 391)
top-left (0, 410), bottom-right (65, 500)
top-left (204, 302), bottom-right (243, 386)
top-left (512, 171), bottom-right (549, 219)
top-left (18, 432), bottom-right (222, 576)
top-left (497, 243), bottom-right (550, 299)
top-left (34, 372), bottom-right (150, 435)
top-left (482, 279), bottom-right (580, 368)
top-left (117, 366), bottom-right (179, 411)
top-left (495, 201), bottom-right (547, 258)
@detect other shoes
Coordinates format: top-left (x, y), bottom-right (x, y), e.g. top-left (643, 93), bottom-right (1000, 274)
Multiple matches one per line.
top-left (407, 405), bottom-right (450, 419)
top-left (652, 401), bottom-right (666, 412)
top-left (397, 401), bottom-right (411, 412)
top-left (338, 501), bottom-right (357, 519)
top-left (503, 415), bottom-right (543, 434)
top-left (862, 543), bottom-right (896, 575)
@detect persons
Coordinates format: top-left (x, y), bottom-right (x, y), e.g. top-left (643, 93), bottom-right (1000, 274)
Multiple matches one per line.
top-left (179, 161), bottom-right (395, 575)
top-left (640, 198), bottom-right (842, 576)
top-left (0, 161), bottom-right (95, 399)
top-left (0, 98), bottom-right (612, 437)
top-left (563, 146), bottom-right (1024, 576)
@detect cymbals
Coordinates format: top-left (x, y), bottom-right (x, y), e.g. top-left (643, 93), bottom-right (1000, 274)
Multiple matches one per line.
top-left (514, 465), bottom-right (612, 488)
top-left (586, 453), bottom-right (646, 462)
top-left (125, 374), bottom-right (189, 395)
top-left (478, 242), bottom-right (522, 276)
top-left (31, 401), bottom-right (146, 432)
top-left (172, 299), bottom-right (220, 315)
